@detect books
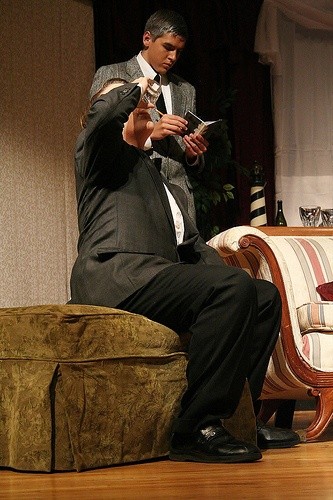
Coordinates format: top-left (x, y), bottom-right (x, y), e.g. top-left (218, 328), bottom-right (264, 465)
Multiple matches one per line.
top-left (175, 110), bottom-right (222, 139)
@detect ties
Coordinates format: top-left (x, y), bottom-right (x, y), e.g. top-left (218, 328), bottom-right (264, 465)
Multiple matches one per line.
top-left (154, 74), bottom-right (167, 118)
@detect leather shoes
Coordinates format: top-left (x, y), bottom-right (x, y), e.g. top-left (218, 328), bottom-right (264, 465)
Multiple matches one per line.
top-left (255, 421), bottom-right (301, 448)
top-left (169, 419), bottom-right (263, 463)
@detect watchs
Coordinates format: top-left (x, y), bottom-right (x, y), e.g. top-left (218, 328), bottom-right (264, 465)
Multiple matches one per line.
top-left (70, 77), bottom-right (300, 464)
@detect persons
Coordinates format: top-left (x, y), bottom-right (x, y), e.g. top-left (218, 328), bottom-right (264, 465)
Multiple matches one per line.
top-left (89, 8), bottom-right (210, 227)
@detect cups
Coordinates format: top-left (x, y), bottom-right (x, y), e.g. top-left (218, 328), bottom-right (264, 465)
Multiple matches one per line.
top-left (321, 208), bottom-right (333, 227)
top-left (135, 79), bottom-right (162, 108)
top-left (298, 205), bottom-right (321, 227)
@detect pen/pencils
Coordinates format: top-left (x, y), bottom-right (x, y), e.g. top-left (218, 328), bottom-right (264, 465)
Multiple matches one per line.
top-left (154, 106), bottom-right (166, 115)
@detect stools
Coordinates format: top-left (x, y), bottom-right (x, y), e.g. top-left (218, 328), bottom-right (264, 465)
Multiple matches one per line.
top-left (1, 305), bottom-right (257, 474)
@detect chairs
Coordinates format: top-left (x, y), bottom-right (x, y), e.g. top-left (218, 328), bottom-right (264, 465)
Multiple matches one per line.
top-left (205, 225), bottom-right (333, 440)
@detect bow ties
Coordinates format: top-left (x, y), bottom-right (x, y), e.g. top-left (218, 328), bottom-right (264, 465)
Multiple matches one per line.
top-left (140, 148), bottom-right (154, 156)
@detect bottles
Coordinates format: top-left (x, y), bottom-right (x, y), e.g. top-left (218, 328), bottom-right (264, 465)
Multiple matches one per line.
top-left (250, 165), bottom-right (268, 225)
top-left (274, 200), bottom-right (287, 227)
